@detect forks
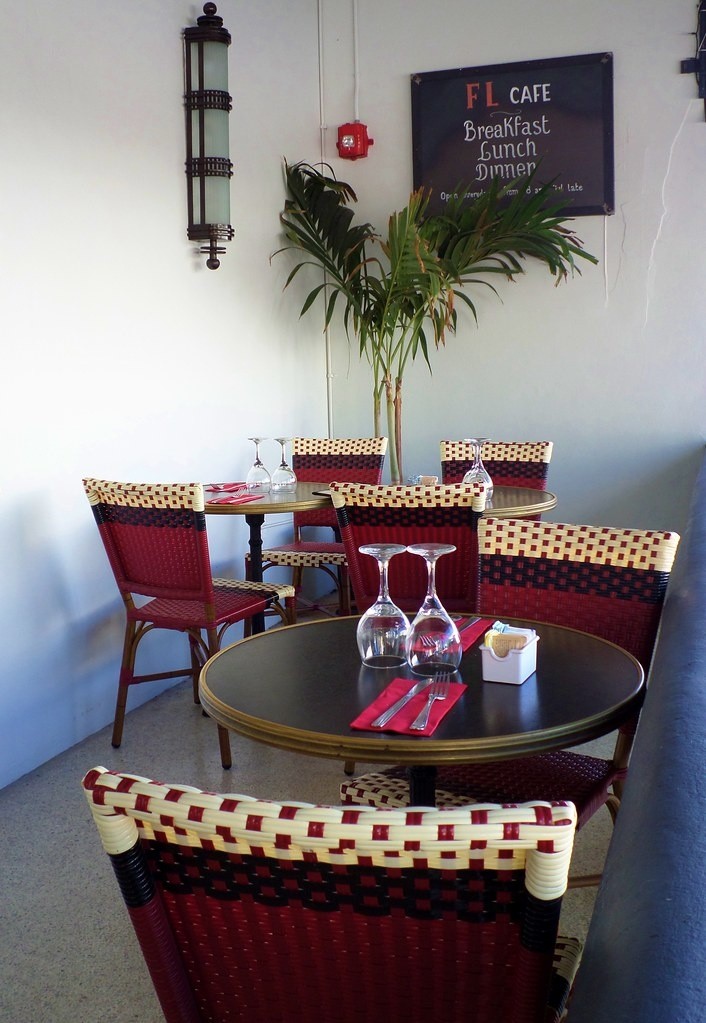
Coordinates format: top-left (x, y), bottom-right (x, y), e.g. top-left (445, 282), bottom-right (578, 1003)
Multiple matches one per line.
top-left (208, 486), bottom-right (245, 504)
top-left (410, 671), bottom-right (451, 730)
top-left (419, 614), bottom-right (463, 646)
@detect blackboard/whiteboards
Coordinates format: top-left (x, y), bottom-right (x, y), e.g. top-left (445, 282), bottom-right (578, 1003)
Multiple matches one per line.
top-left (408, 52), bottom-right (616, 222)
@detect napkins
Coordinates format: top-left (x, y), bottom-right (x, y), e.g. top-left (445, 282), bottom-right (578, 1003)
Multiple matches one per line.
top-left (205, 484), bottom-right (260, 492)
top-left (206, 493), bottom-right (265, 505)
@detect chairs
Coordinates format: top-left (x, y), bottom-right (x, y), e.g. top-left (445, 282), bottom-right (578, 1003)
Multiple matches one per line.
top-left (243, 436), bottom-right (389, 637)
top-left (82, 766), bottom-right (582, 1023)
top-left (339, 516), bottom-right (680, 835)
top-left (440, 440), bottom-right (553, 521)
top-left (82, 477), bottom-right (296, 770)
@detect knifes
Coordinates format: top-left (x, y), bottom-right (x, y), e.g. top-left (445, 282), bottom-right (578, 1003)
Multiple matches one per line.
top-left (434, 616), bottom-right (482, 654)
top-left (370, 678), bottom-right (434, 728)
top-left (217, 484), bottom-right (248, 492)
top-left (225, 494), bottom-right (265, 502)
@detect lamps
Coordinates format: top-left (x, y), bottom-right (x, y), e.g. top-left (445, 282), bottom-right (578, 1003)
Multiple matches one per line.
top-left (181, 2), bottom-right (234, 271)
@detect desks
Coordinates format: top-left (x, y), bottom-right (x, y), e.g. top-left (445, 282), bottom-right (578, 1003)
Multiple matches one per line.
top-left (200, 482), bottom-right (333, 637)
top-left (440, 482), bottom-right (558, 520)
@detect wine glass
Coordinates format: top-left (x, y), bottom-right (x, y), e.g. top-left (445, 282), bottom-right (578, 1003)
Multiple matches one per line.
top-left (357, 539), bottom-right (410, 678)
top-left (406, 543), bottom-right (463, 678)
top-left (245, 434), bottom-right (298, 494)
top-left (460, 437), bottom-right (496, 502)
